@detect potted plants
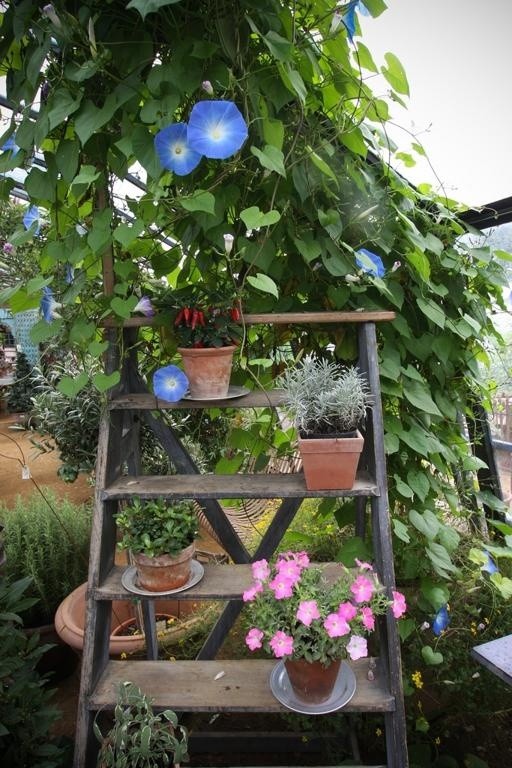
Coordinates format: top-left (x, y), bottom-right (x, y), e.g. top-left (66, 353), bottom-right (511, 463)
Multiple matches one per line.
top-left (110, 491), bottom-right (200, 592)
top-left (156, 284), bottom-right (246, 396)
top-left (266, 342), bottom-right (379, 492)
top-left (1, 483), bottom-right (96, 675)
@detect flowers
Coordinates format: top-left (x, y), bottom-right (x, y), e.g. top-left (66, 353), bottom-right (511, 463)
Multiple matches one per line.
top-left (235, 544), bottom-right (410, 667)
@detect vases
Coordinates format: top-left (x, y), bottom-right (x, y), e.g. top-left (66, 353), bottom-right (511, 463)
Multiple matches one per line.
top-left (285, 650), bottom-right (341, 703)
top-left (53, 562), bottom-right (228, 661)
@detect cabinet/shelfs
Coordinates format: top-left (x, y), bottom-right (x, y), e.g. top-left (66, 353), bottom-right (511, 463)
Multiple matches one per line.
top-left (72, 291), bottom-right (424, 768)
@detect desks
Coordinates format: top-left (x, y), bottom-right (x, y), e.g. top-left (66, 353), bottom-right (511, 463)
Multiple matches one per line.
top-left (468, 631), bottom-right (511, 688)
